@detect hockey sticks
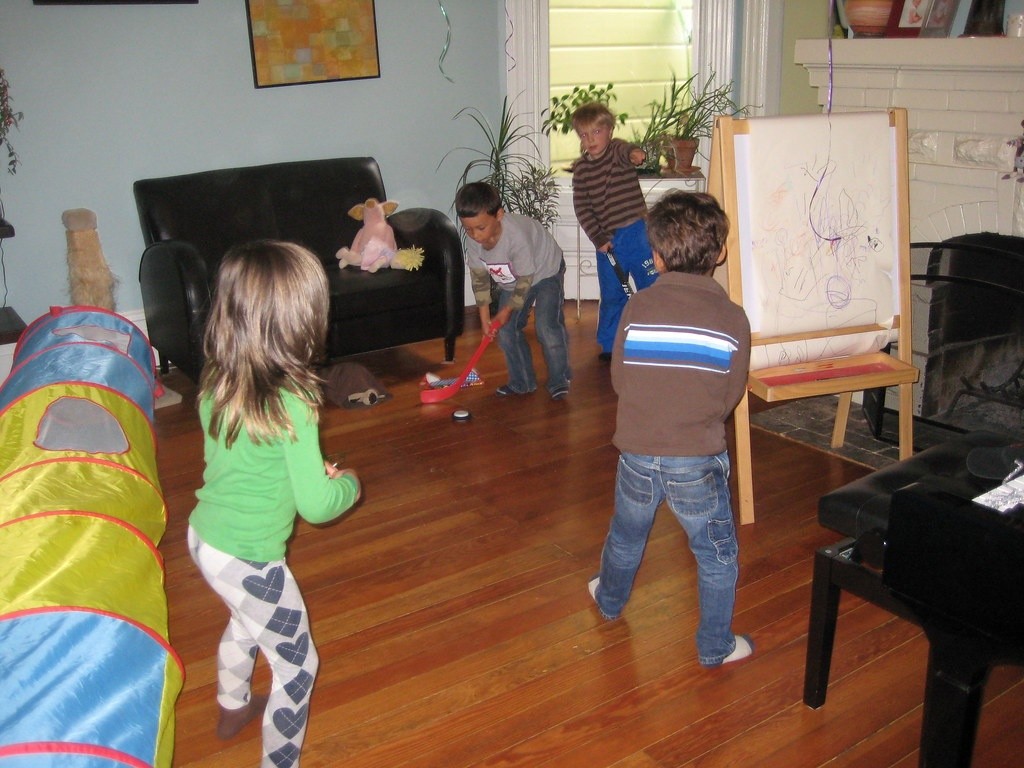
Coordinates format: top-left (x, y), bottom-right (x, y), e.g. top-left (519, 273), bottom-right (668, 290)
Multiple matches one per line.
top-left (418, 321), bottom-right (504, 403)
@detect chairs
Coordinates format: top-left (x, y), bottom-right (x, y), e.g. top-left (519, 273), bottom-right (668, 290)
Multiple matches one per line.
top-left (804, 429), bottom-right (1024, 768)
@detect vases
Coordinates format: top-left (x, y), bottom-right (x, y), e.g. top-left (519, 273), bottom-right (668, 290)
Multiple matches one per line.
top-left (844, 0), bottom-right (894, 38)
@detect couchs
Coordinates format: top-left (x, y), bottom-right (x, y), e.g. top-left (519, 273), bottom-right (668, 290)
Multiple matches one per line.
top-left (133, 157), bottom-right (465, 384)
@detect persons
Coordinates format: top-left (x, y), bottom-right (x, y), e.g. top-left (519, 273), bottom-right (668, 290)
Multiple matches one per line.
top-left (587, 189), bottom-right (754, 666)
top-left (909, 0), bottom-right (924, 24)
top-left (187, 239), bottom-right (363, 767)
top-left (571, 103), bottom-right (659, 362)
top-left (455, 180), bottom-right (572, 399)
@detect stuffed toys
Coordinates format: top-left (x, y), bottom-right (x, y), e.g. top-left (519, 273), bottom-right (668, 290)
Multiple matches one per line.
top-left (335, 198), bottom-right (425, 273)
top-left (321, 363), bottom-right (388, 407)
top-left (1001, 119), bottom-right (1024, 182)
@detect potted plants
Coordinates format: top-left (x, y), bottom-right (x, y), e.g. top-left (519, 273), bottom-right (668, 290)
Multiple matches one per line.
top-left (636, 71), bottom-right (764, 181)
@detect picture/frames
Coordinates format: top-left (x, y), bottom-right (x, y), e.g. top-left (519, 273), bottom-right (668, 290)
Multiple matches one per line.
top-left (963, 0), bottom-right (1005, 35)
top-left (884, 0), bottom-right (961, 39)
top-left (246, 0), bottom-right (380, 89)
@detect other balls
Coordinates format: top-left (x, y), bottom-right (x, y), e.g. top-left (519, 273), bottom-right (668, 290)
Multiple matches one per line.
top-left (452, 410), bottom-right (472, 421)
